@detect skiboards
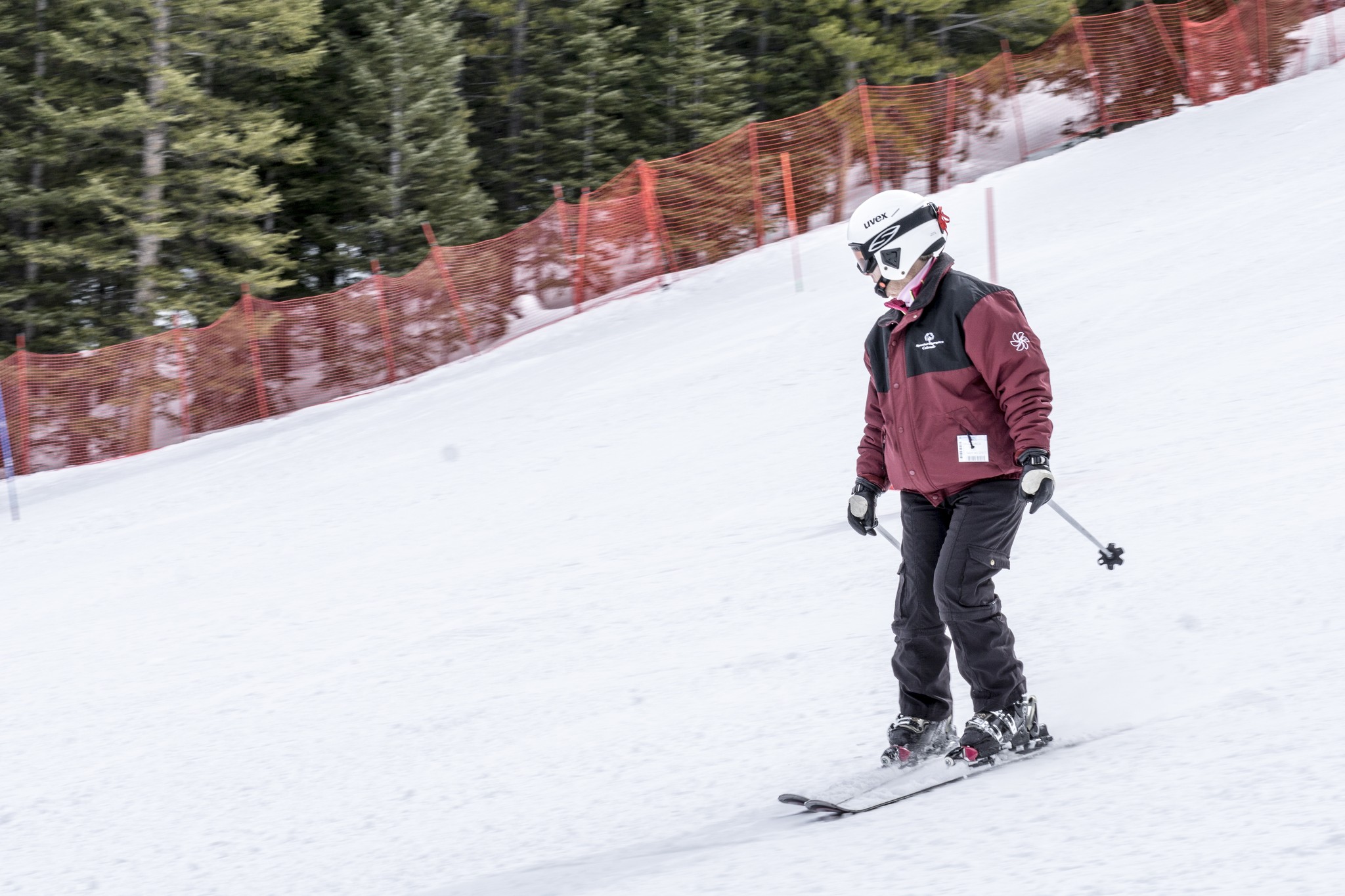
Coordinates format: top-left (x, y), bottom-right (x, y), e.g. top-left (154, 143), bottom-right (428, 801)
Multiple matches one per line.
top-left (778, 719), bottom-right (1146, 814)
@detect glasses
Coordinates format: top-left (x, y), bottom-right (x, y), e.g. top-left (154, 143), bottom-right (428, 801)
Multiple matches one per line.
top-left (848, 242), bottom-right (877, 274)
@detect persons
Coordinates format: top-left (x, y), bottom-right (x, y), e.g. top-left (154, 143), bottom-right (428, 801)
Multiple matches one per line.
top-left (847, 189), bottom-right (1060, 759)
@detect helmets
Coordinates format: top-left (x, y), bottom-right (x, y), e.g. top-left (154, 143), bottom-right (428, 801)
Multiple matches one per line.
top-left (849, 189), bottom-right (951, 282)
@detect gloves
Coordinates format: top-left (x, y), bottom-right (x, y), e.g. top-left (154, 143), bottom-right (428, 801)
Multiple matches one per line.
top-left (1015, 448), bottom-right (1055, 513)
top-left (848, 478), bottom-right (881, 536)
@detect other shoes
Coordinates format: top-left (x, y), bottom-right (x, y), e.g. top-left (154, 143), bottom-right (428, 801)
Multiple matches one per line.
top-left (890, 709), bottom-right (955, 749)
top-left (959, 699), bottom-right (1024, 746)
